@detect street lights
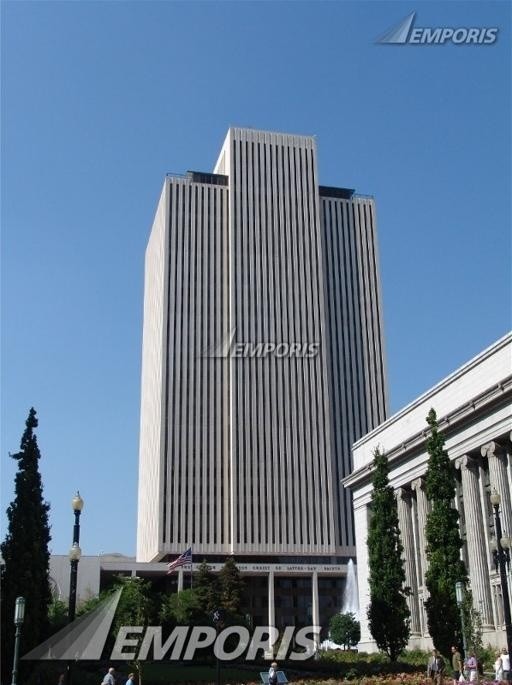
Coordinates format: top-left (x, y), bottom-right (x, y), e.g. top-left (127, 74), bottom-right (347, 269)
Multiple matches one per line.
top-left (11, 596), bottom-right (26, 685)
top-left (68, 490), bottom-right (84, 623)
top-left (490, 488), bottom-right (512, 685)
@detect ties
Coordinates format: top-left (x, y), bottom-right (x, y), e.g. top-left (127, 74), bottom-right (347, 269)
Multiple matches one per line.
top-left (434, 658), bottom-right (436, 671)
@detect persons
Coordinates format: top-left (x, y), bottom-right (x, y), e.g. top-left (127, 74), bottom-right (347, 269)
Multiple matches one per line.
top-left (450, 646), bottom-right (465, 685)
top-left (125, 673), bottom-right (135, 685)
top-left (463, 649), bottom-right (479, 685)
top-left (58, 666), bottom-right (72, 685)
top-left (268, 662), bottom-right (278, 685)
top-left (101, 667), bottom-right (116, 685)
top-left (501, 647), bottom-right (512, 681)
top-left (428, 649), bottom-right (447, 685)
top-left (494, 651), bottom-right (504, 681)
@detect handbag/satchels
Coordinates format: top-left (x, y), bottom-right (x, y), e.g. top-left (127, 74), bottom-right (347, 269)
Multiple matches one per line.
top-left (269, 677), bottom-right (277, 684)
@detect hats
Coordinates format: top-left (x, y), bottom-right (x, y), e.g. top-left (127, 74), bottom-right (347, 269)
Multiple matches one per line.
top-left (271, 662), bottom-right (277, 667)
top-left (109, 668), bottom-right (115, 673)
top-left (128, 673), bottom-right (134, 678)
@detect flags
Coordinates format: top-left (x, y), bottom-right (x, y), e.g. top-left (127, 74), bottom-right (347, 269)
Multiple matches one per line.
top-left (166, 547), bottom-right (192, 574)
